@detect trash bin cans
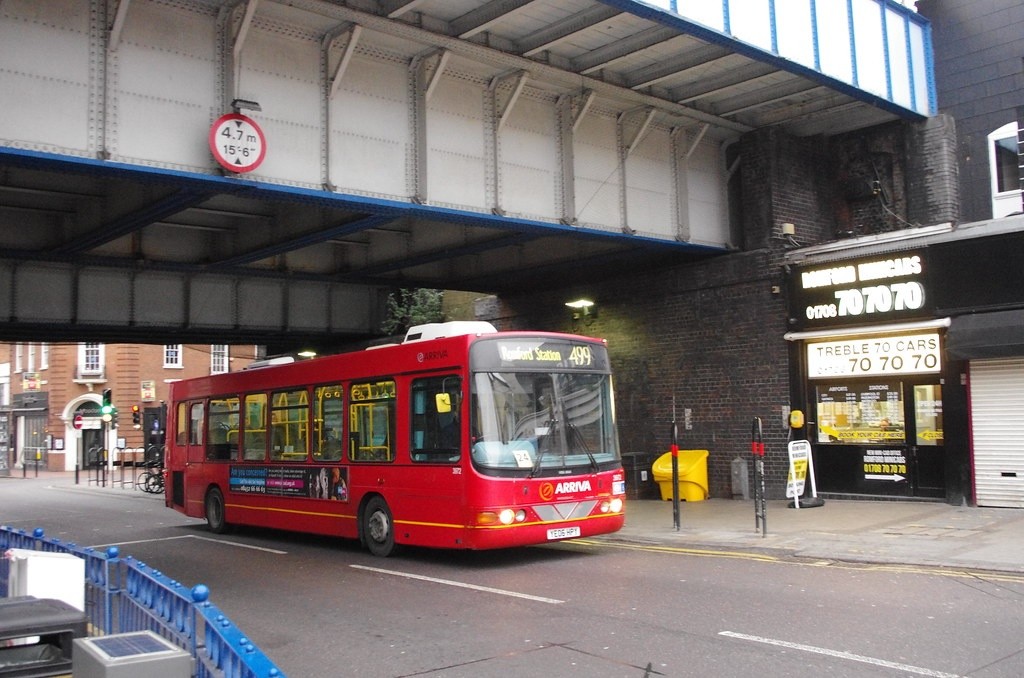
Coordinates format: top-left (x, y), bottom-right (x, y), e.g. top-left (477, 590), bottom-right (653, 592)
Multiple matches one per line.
top-left (620, 451), bottom-right (652, 498)
top-left (653, 449), bottom-right (710, 502)
top-left (0, 596), bottom-right (87, 678)
top-left (7, 548), bottom-right (85, 646)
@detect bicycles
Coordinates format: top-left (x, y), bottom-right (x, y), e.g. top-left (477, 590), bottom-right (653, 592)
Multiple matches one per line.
top-left (137, 470), bottom-right (165, 494)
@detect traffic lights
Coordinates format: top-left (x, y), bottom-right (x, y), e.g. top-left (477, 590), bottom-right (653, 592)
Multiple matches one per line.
top-left (131, 404), bottom-right (140, 424)
top-left (101, 387), bottom-right (112, 422)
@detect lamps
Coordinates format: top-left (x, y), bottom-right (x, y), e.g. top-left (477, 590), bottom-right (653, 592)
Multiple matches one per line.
top-left (564, 297), bottom-right (596, 320)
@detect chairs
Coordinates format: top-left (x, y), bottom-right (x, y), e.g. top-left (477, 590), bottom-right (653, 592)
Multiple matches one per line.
top-left (178, 421), bottom-right (379, 461)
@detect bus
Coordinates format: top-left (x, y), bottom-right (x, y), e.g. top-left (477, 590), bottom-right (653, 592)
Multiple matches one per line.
top-left (159, 316), bottom-right (631, 560)
